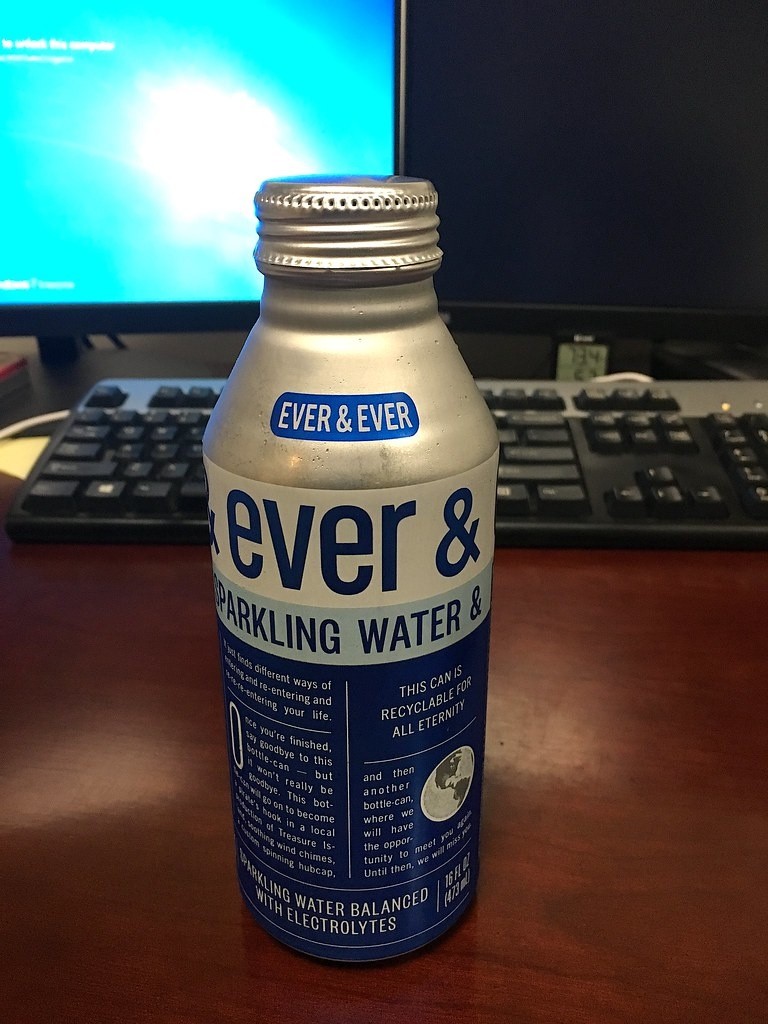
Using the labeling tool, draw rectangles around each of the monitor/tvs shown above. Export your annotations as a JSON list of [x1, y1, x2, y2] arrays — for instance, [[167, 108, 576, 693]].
[[0, 1, 768, 335]]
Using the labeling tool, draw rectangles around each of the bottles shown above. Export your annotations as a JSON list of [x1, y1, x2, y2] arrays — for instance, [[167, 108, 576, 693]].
[[199, 171, 502, 972]]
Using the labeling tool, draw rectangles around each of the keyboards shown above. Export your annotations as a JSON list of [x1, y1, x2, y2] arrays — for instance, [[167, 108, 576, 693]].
[[9, 374, 768, 549]]
